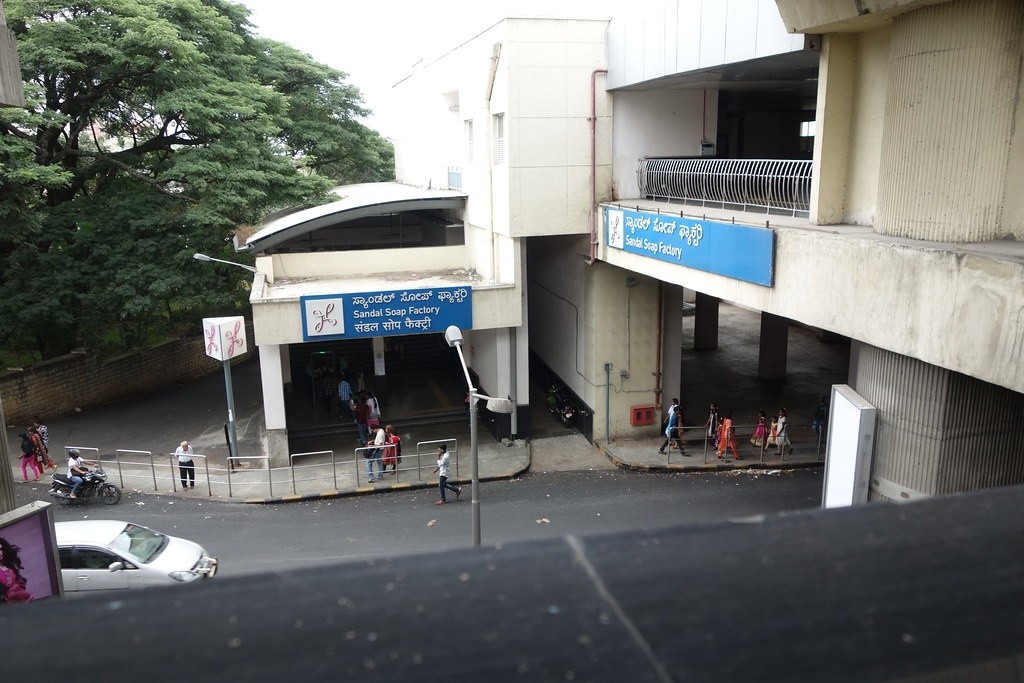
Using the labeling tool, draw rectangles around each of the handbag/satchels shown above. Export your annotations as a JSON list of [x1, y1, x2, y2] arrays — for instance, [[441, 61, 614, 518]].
[[71, 466, 88, 476], [367, 419, 380, 426], [362, 443, 377, 458]]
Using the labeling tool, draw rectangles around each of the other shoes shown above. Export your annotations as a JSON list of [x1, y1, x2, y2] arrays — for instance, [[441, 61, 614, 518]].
[[191, 486, 194, 488], [183, 488, 188, 491], [367, 478, 374, 483], [34, 478, 39, 481], [735, 456, 744, 460], [789, 448, 793, 455], [435, 500, 446, 504], [377, 475, 383, 480], [391, 472, 395, 475], [54, 465, 58, 472], [682, 454, 691, 457], [457, 487, 461, 498], [718, 456, 725, 459], [775, 452, 781, 455], [658, 451, 666, 455], [19, 479, 28, 483], [70, 494, 76, 498]]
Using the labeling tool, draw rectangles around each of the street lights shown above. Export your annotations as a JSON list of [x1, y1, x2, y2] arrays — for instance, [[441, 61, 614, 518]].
[[446, 324, 514, 548]]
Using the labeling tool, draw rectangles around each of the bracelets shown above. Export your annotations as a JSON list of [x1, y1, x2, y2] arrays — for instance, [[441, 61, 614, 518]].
[[93, 463, 95, 466]]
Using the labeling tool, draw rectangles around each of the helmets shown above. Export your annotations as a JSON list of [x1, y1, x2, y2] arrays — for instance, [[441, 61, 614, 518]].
[[69, 449, 80, 459]]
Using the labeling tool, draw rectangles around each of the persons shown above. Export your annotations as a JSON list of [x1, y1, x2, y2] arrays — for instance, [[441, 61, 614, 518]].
[[17, 432, 40, 484], [28, 427, 58, 474], [658, 398, 793, 459], [320, 369, 402, 483], [32, 419, 51, 470], [67, 449, 100, 498], [174, 441, 195, 491], [433, 444, 461, 505], [0, 538, 40, 604], [812, 394, 828, 446]]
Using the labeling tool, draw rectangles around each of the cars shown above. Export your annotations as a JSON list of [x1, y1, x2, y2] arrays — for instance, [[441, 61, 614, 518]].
[[54, 520, 217, 594]]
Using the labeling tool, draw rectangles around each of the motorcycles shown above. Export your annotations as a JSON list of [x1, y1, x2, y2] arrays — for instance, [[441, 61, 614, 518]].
[[547, 384, 575, 427], [49, 464, 121, 505]]
